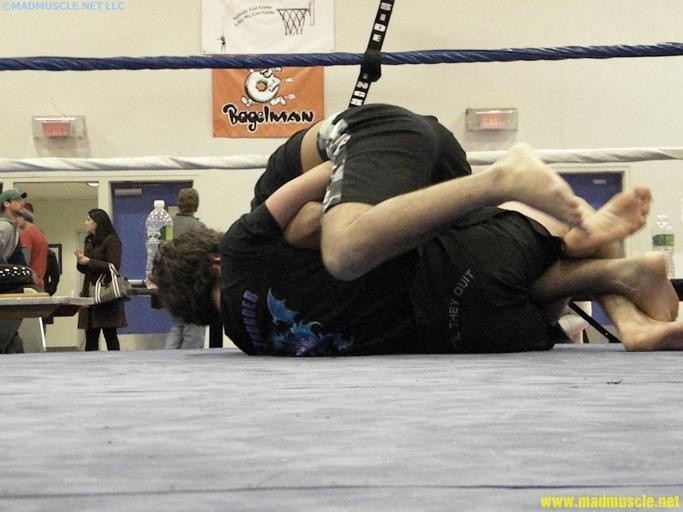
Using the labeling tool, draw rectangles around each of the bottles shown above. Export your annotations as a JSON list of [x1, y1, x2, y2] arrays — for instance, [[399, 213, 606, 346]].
[[651, 214, 674, 280], [143, 199, 174, 290]]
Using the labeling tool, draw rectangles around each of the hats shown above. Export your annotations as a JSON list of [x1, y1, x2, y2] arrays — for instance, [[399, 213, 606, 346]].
[[0, 190, 27, 205], [19, 204, 34, 223]]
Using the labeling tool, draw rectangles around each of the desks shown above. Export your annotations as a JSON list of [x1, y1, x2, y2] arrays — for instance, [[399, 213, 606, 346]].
[[127, 287, 223, 349], [1, 294, 92, 355]]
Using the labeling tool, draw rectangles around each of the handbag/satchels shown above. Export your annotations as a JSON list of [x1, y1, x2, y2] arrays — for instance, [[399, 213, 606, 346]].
[[88, 262, 131, 306]]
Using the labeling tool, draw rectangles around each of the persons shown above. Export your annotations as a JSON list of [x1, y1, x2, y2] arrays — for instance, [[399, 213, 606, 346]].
[[14, 202, 48, 293], [41, 247, 59, 335], [73, 208, 122, 351], [161, 185, 209, 350], [147, 159, 679, 357], [248, 101, 682, 353], [559, 300, 592, 344], [0, 188, 27, 355]]
